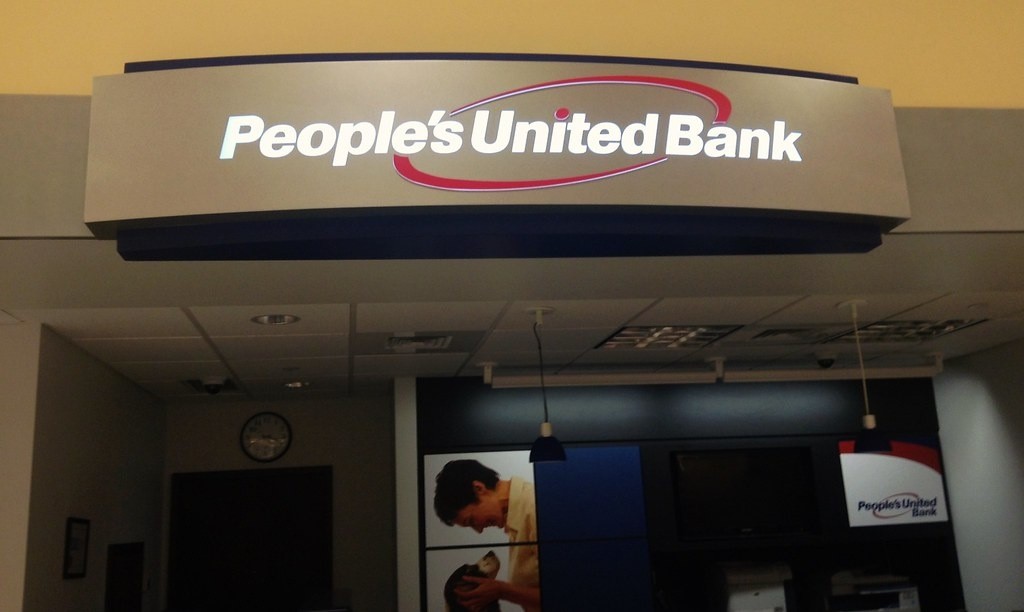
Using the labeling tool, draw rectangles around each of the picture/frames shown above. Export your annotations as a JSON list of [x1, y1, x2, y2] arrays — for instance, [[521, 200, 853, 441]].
[[62, 517, 90, 580]]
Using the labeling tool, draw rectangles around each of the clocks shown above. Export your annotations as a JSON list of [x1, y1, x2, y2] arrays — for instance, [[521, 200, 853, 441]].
[[240, 412, 292, 463]]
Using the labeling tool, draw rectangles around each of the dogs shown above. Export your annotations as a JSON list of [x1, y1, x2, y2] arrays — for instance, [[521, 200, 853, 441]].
[[444, 550, 501, 612]]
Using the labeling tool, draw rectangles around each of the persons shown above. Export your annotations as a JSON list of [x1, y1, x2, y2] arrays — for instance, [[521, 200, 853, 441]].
[[433, 460, 540, 611]]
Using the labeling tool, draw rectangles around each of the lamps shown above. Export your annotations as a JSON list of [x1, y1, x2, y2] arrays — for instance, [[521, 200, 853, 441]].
[[839, 299, 892, 454], [526, 306, 568, 464]]
